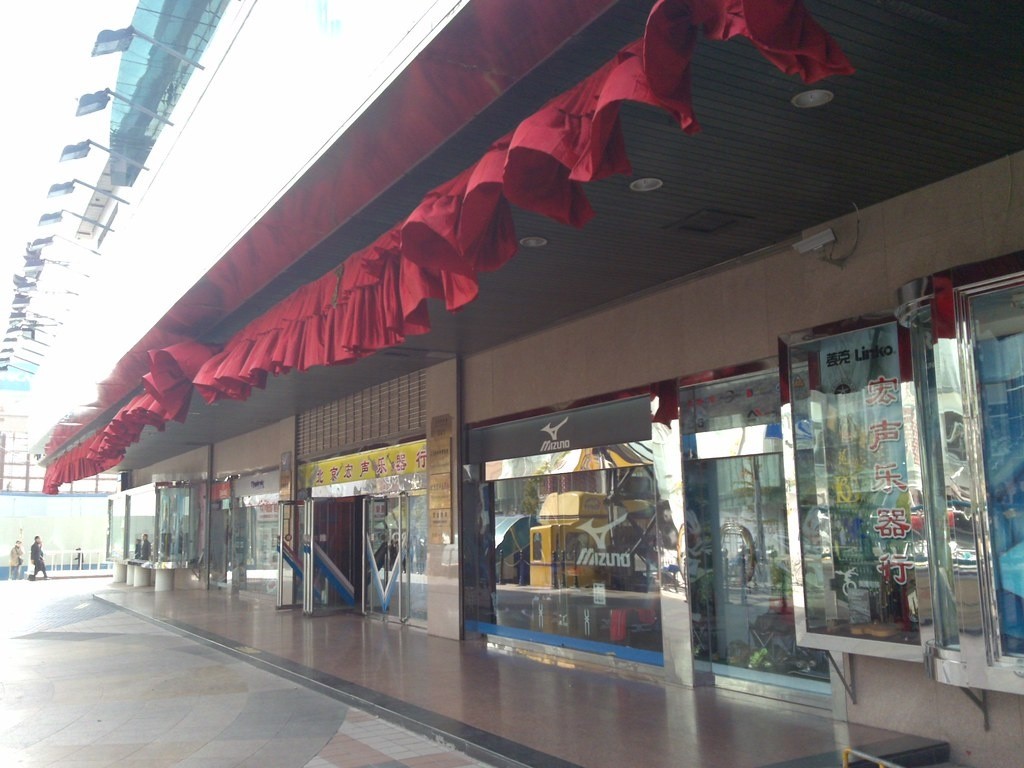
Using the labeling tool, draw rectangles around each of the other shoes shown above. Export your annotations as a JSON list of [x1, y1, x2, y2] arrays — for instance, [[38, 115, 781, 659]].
[[44, 577, 51, 580]]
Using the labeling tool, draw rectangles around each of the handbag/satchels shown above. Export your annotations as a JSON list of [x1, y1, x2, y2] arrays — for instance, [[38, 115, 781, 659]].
[[19, 558, 23, 565]]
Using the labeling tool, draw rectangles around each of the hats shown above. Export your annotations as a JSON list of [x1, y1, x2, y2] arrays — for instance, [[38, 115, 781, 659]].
[[76, 548, 81, 550]]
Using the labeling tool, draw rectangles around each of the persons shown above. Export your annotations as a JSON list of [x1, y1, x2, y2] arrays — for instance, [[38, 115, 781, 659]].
[[9, 540, 24, 580], [134, 539, 142, 559], [75, 548, 84, 570], [142, 534, 152, 561], [31, 536, 50, 580]]
